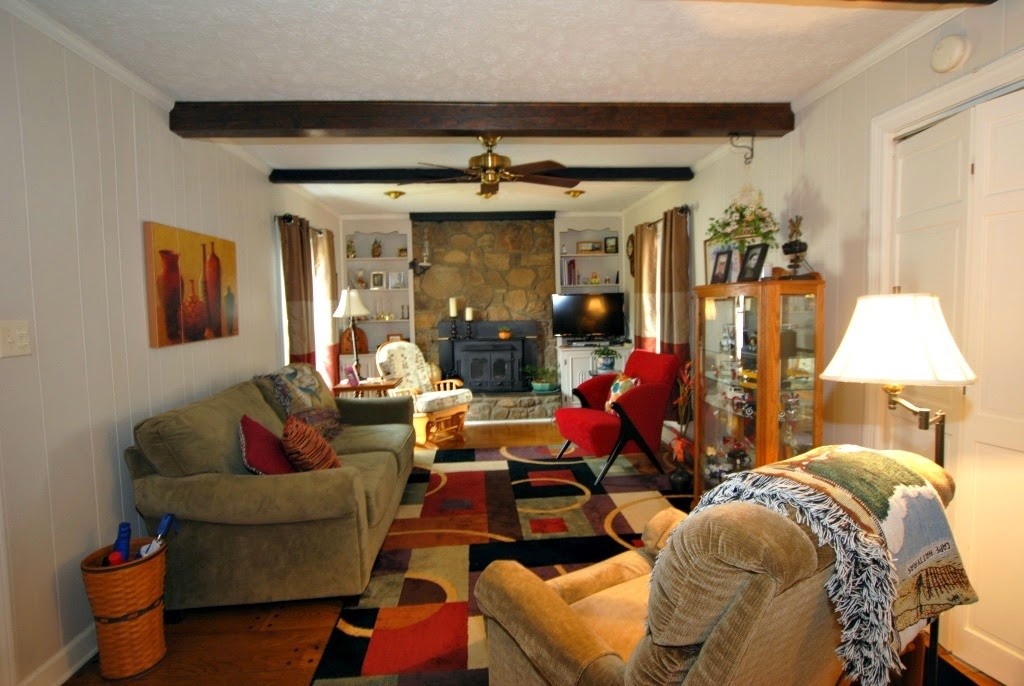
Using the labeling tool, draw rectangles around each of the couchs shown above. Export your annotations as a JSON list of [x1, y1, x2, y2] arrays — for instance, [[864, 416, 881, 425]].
[[132, 365, 416, 612]]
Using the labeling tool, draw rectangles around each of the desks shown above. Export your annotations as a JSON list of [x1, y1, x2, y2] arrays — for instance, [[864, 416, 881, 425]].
[[333, 377, 403, 398]]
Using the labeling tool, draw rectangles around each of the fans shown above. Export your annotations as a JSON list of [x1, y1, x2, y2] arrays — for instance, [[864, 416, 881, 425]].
[[417, 134, 579, 196]]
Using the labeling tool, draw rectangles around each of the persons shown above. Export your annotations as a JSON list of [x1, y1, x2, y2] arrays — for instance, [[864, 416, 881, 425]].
[[712, 252, 760, 283]]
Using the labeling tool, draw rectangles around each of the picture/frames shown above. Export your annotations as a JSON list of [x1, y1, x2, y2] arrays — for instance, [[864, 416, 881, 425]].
[[704, 239, 769, 284]]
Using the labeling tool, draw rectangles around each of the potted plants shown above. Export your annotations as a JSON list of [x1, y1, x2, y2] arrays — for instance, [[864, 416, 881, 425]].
[[594, 345, 616, 369], [527, 367, 559, 391], [498, 321, 510, 339]]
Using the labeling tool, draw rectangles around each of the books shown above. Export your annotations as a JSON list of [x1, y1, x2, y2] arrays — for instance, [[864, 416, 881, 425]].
[[561, 257, 577, 285]]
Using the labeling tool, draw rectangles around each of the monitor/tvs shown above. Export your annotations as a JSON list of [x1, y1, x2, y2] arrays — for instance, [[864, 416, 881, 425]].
[[551, 294, 625, 342]]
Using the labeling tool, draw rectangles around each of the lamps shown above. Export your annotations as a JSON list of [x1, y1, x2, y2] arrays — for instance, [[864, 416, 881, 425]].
[[333, 289, 370, 380], [820, 286, 978, 685]]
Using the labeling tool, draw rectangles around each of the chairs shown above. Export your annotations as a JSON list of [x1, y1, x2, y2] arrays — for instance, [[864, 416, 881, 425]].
[[554, 350, 676, 479], [476, 445, 954, 686], [376, 341, 471, 450]]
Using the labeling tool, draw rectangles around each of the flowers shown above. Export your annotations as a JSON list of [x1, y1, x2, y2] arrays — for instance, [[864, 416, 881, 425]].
[[708, 186, 781, 246]]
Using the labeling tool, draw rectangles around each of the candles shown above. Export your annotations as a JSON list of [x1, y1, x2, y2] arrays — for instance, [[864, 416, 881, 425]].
[[449, 297, 457, 316], [465, 307, 473, 320]]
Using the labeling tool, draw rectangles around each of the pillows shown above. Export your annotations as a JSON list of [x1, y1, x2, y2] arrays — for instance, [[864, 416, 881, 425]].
[[240, 363, 340, 475]]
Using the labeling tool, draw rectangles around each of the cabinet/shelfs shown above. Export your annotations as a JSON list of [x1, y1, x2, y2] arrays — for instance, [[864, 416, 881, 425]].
[[340, 217, 415, 400], [692, 275, 825, 509], [556, 213, 634, 407]]
